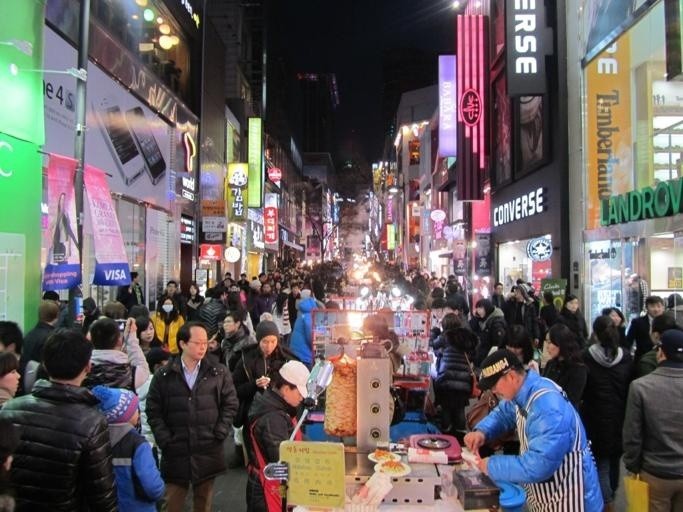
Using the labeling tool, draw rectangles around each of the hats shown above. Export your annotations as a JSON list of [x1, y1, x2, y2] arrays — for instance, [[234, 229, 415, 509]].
[[279, 359, 311, 400], [256, 320, 279, 341], [476, 350, 520, 390], [653, 329, 683, 353], [92, 384, 139, 424]]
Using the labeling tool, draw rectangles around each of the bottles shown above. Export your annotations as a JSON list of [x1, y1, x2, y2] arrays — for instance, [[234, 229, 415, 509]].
[[404, 329, 424, 336], [303, 359, 333, 409], [393, 306, 404, 327], [404, 314, 411, 328]]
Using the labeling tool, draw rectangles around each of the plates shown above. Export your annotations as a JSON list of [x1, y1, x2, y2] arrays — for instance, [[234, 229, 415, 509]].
[[373, 462, 412, 479], [366, 452, 401, 464]]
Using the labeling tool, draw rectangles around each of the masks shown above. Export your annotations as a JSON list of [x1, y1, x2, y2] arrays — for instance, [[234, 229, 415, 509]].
[[162, 304, 173, 312]]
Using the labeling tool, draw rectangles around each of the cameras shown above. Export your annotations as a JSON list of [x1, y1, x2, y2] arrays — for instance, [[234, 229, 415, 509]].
[[114, 319, 127, 332]]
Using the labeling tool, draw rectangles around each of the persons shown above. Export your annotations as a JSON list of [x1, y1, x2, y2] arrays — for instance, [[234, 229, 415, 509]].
[[1, 251, 683, 511]]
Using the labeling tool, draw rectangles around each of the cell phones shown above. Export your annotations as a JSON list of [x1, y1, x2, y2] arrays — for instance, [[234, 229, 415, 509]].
[[91, 96, 145, 186], [125, 107, 166, 185]]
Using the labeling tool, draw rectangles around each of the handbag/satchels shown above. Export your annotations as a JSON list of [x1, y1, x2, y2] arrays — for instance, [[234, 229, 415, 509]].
[[623, 475, 649, 512], [466, 390, 502, 450]]
